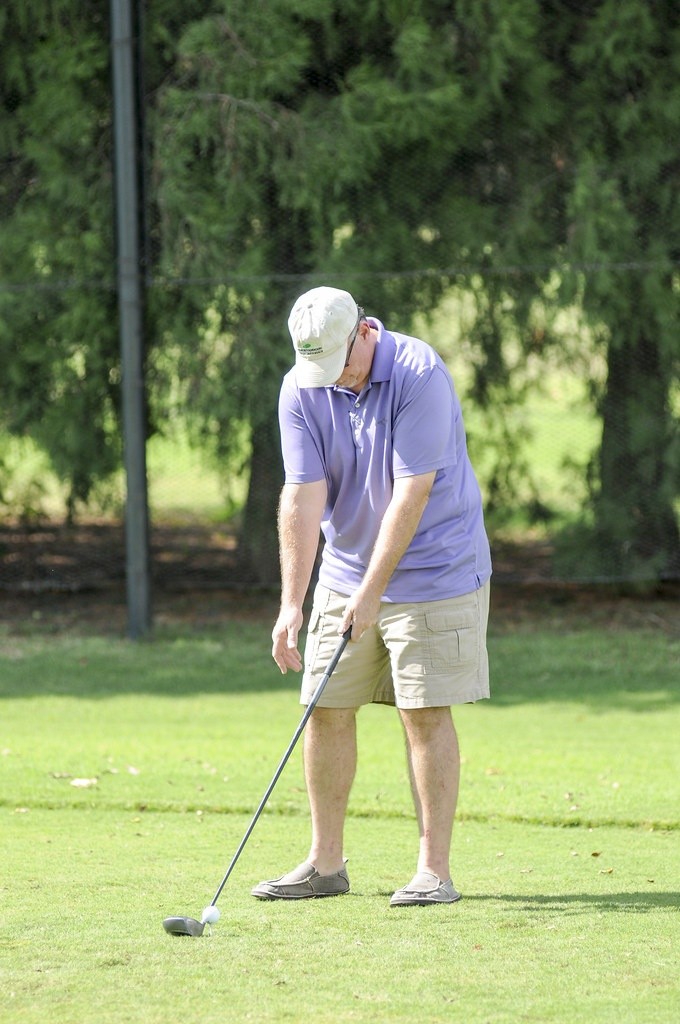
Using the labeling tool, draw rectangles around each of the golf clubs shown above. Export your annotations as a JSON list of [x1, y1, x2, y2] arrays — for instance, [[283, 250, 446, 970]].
[[161, 623, 354, 939]]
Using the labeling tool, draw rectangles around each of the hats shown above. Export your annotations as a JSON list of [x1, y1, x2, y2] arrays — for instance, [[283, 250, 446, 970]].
[[287, 286, 359, 389]]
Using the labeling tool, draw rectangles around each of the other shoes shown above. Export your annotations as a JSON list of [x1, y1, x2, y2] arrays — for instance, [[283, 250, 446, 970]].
[[250, 859, 349, 898], [389, 872, 462, 907]]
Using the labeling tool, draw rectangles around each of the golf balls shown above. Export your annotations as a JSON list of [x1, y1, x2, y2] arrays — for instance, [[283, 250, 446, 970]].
[[202, 906, 220, 923]]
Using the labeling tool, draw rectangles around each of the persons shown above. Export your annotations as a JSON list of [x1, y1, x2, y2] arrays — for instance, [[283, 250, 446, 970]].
[[251, 286, 493, 908]]
